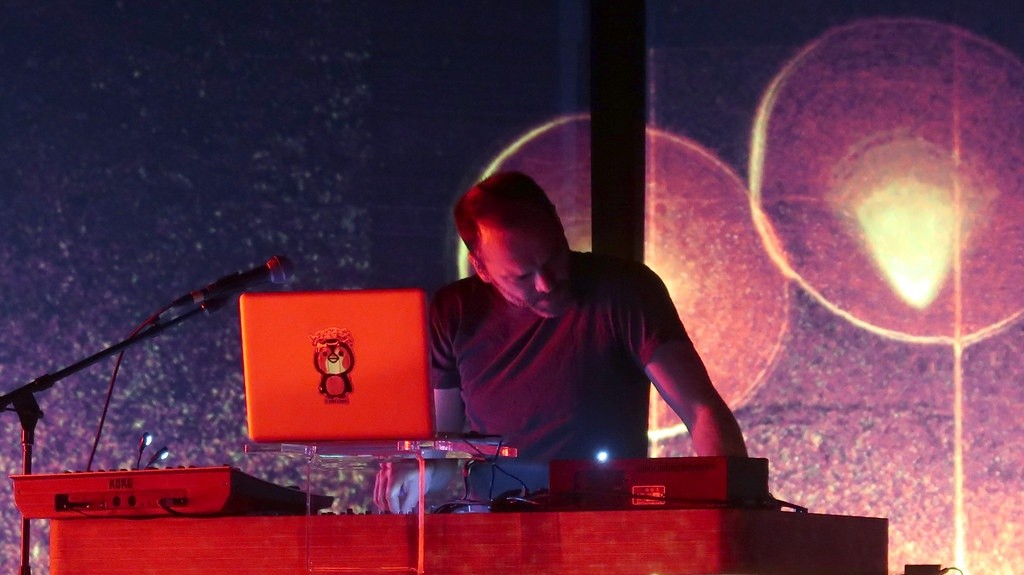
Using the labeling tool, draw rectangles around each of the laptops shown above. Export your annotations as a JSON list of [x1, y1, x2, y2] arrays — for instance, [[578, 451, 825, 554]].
[[239, 288, 511, 447]]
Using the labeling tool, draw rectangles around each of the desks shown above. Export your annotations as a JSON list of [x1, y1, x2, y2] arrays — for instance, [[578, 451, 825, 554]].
[[49, 509, 890, 575]]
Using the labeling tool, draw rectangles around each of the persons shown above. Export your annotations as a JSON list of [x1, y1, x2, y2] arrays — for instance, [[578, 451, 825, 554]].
[[373, 171, 774, 513]]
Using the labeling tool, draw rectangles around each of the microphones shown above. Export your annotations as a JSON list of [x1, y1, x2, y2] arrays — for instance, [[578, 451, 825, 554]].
[[177, 255, 294, 310]]
